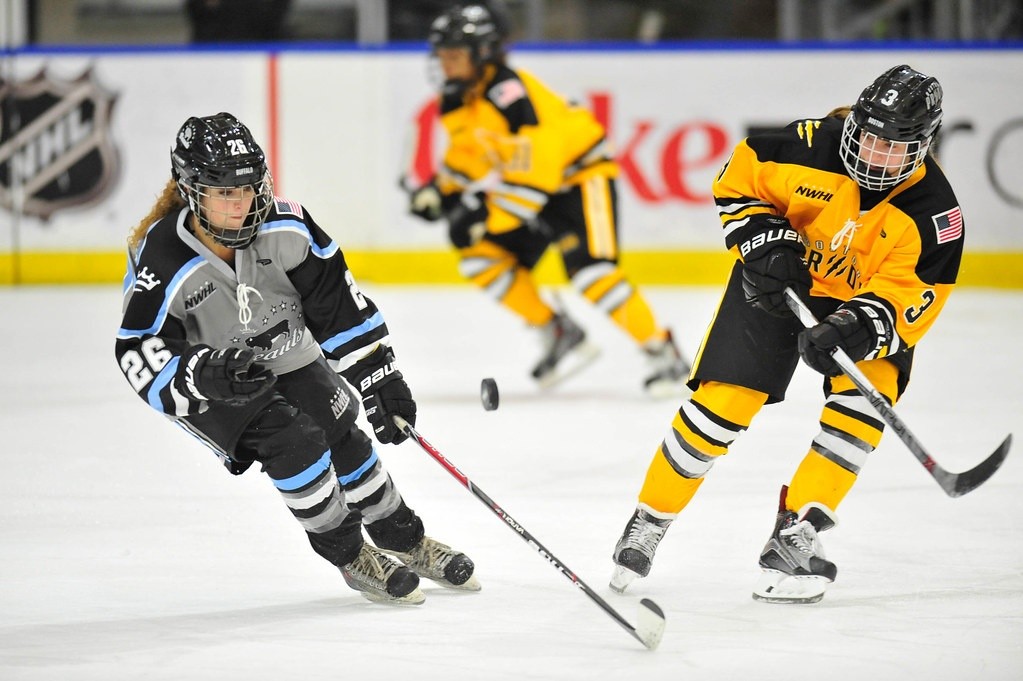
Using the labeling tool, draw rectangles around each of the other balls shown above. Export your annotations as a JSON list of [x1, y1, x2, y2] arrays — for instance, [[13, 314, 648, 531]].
[[478, 375, 505, 410]]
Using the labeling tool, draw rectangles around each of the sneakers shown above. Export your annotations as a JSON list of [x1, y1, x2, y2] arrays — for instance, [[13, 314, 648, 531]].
[[609, 502, 677, 593], [644, 329, 690, 399], [752, 501, 840, 605], [338, 541, 425, 602], [396, 535, 481, 591], [530, 312, 592, 391]]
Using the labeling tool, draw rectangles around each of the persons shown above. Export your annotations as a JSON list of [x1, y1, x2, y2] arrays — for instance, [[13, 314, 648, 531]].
[[400, 7, 692, 400], [611, 65, 964, 605], [116, 113, 482, 603]]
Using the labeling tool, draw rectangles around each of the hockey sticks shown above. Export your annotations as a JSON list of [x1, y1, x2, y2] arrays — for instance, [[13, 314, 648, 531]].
[[393, 418, 669, 652], [787, 282, 1014, 497]]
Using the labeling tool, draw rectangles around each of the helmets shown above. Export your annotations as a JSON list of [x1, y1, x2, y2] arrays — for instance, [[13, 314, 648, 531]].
[[170, 112, 274, 250], [428, 4, 509, 95], [838, 64, 943, 191]]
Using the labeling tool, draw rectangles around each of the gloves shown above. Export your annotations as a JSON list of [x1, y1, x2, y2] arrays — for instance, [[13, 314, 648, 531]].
[[736, 214, 813, 318], [449, 192, 489, 248], [411, 183, 444, 220], [346, 343, 417, 445], [798, 292, 908, 378], [176, 344, 278, 401]]
